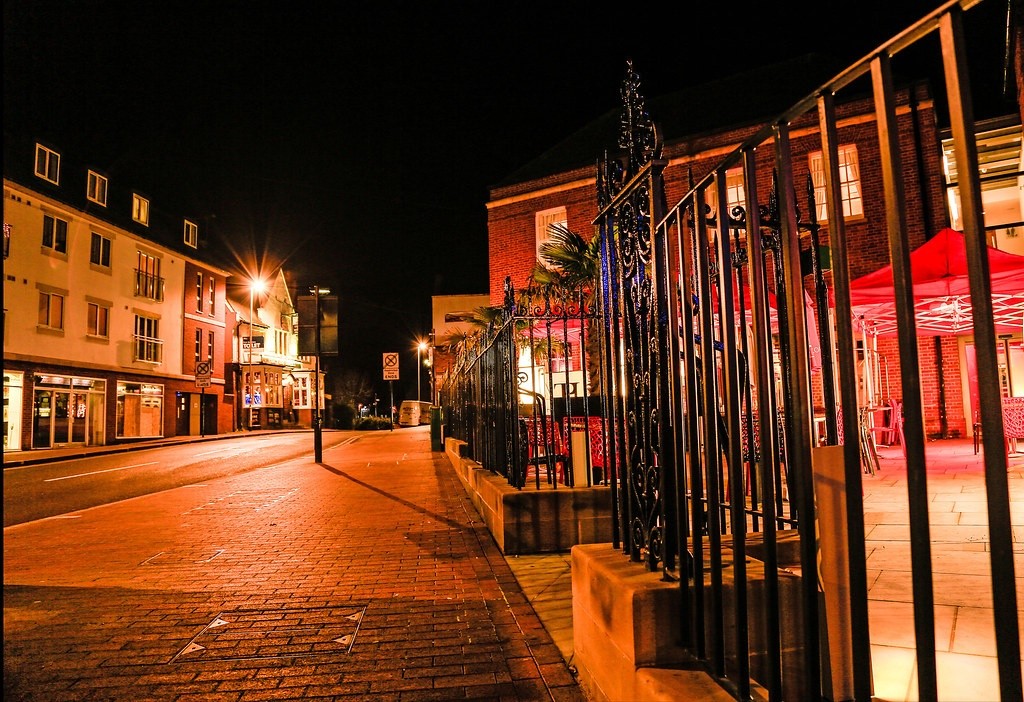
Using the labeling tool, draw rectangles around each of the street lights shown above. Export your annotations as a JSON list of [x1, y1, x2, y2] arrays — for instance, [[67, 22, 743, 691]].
[[417, 341, 428, 400], [248, 279, 267, 431], [309, 284, 331, 463]]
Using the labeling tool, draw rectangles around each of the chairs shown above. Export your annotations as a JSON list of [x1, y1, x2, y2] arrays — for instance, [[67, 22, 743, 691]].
[[972, 409, 983, 454], [1002, 397, 1024, 449], [528, 412, 623, 484], [869, 399, 904, 452]]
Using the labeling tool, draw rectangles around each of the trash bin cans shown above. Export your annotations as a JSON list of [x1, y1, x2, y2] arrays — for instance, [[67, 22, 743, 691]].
[[420, 401, 433, 425], [399, 400, 421, 426]]
